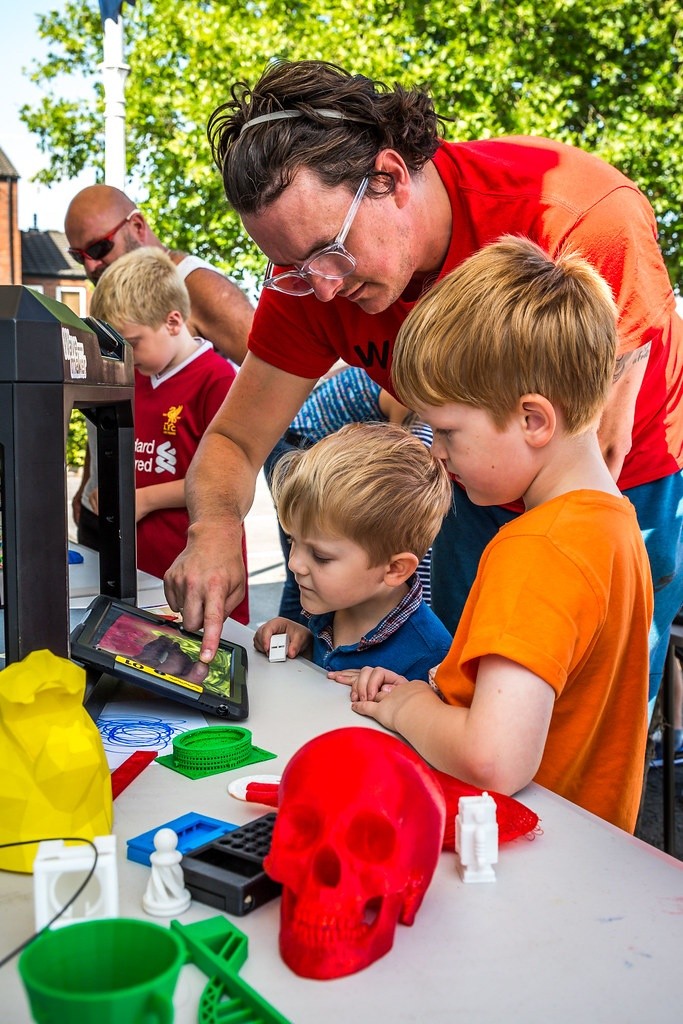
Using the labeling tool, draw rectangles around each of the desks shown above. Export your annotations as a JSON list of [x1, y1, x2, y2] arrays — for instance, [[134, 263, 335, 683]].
[[0, 541, 683, 1023]]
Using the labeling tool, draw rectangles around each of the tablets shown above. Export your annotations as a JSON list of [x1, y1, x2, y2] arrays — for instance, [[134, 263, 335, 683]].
[[70, 595, 248, 722]]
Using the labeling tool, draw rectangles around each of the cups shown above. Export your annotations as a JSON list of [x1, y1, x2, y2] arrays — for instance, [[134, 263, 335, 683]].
[[18, 918, 188, 1024]]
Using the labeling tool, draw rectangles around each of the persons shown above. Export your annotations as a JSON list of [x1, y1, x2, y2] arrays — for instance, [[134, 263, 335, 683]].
[[324, 231, 657, 836], [252, 420, 455, 685], [63, 184, 257, 552], [86, 244, 251, 629], [163, 57, 683, 779]]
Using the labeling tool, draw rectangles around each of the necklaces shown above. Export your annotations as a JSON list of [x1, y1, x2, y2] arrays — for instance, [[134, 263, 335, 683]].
[[166, 248, 171, 255]]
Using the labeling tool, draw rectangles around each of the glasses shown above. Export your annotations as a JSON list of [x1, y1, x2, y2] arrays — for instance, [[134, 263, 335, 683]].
[[263, 170, 372, 297], [67, 210, 142, 265]]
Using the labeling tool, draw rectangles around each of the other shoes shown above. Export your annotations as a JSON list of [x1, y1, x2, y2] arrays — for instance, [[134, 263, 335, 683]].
[[647, 725, 683, 767]]
